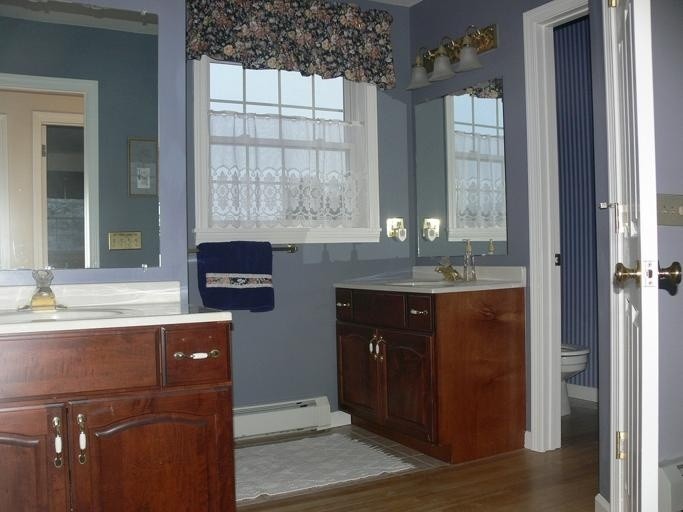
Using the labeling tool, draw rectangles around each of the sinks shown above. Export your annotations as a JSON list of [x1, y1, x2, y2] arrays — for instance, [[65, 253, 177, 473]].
[[384, 280, 460, 291], [0, 308, 125, 328]]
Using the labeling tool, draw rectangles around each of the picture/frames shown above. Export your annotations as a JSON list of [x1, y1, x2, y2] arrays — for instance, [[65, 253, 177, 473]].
[[126, 137, 157, 198]]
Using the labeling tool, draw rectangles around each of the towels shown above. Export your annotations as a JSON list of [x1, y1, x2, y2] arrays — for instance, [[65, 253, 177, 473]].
[[197, 241, 275, 314]]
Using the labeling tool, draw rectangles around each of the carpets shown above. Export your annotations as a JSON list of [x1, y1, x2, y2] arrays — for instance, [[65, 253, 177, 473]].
[[235, 435, 413, 505]]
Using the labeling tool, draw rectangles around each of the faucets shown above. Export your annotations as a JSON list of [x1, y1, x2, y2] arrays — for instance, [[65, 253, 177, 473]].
[[29, 269, 58, 311], [434, 263, 462, 282]]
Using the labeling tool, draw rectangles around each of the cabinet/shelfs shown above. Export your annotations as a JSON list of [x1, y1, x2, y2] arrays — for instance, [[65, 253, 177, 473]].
[[333, 266, 526, 464], [0, 313, 235, 511]]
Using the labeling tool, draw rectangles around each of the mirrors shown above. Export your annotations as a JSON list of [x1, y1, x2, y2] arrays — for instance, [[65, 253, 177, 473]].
[[1, 74, 99, 270], [412, 80, 507, 255]]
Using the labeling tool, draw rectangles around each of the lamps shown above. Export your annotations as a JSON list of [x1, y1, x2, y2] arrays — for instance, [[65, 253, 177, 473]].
[[454, 25, 484, 72], [428, 35, 459, 83], [406, 45, 431, 90]]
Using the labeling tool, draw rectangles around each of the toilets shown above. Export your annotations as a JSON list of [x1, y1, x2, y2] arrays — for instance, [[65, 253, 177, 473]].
[[560, 343, 590, 417]]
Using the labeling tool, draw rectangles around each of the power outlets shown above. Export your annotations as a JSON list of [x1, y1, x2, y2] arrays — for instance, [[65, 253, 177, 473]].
[[422, 218, 438, 238], [109, 232, 143, 250], [386, 217, 405, 238], [657, 194, 683, 226]]
